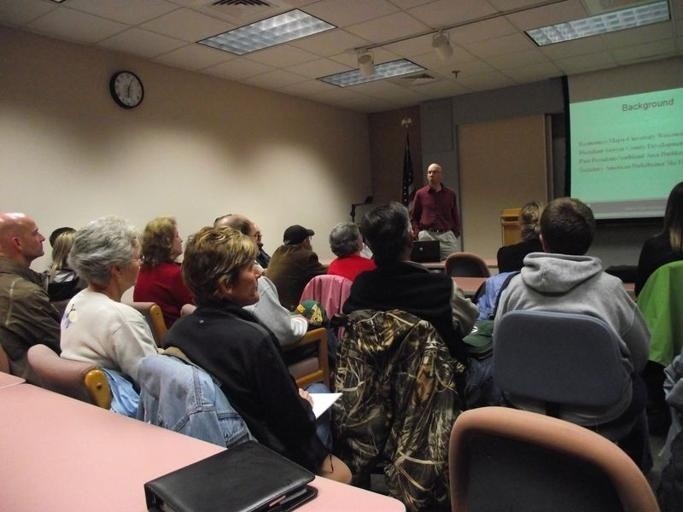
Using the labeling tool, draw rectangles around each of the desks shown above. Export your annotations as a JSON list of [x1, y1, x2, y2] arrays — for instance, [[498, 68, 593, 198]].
[[0, 371, 26, 389], [0, 383, 406, 512]]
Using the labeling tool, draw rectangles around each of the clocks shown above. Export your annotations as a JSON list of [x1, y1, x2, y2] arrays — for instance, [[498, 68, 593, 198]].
[[110, 70, 144, 109]]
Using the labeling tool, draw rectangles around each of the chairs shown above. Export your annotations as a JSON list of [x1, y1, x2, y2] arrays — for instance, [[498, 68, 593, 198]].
[[28, 252, 659, 512]]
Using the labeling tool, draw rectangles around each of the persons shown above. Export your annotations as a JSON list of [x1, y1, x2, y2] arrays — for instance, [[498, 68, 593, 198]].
[[0, 213, 194, 393], [519, 199, 550, 239], [216, 201, 480, 388], [408, 162, 461, 260], [489, 197, 654, 476], [633, 180, 683, 300], [160, 224, 331, 475]]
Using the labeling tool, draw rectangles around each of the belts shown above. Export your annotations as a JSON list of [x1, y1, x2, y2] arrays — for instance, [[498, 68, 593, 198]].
[[426, 227, 449, 233]]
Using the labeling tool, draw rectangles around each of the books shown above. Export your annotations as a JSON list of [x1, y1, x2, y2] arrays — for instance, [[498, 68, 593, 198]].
[[141, 437, 317, 511]]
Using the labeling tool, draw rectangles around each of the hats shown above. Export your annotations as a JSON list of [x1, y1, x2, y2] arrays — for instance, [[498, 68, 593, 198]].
[[462, 319, 494, 359], [291, 300, 328, 323], [284, 225, 314, 245]]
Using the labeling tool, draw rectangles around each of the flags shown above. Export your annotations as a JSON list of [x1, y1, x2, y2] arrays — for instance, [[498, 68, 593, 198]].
[[401, 127, 416, 207]]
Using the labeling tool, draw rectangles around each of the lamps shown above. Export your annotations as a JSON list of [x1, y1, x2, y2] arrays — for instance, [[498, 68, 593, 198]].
[[432, 26, 452, 60], [356, 48, 375, 77]]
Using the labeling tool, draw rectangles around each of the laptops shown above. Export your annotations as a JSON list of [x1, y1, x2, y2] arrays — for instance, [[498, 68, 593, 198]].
[[412, 240, 446, 263]]
